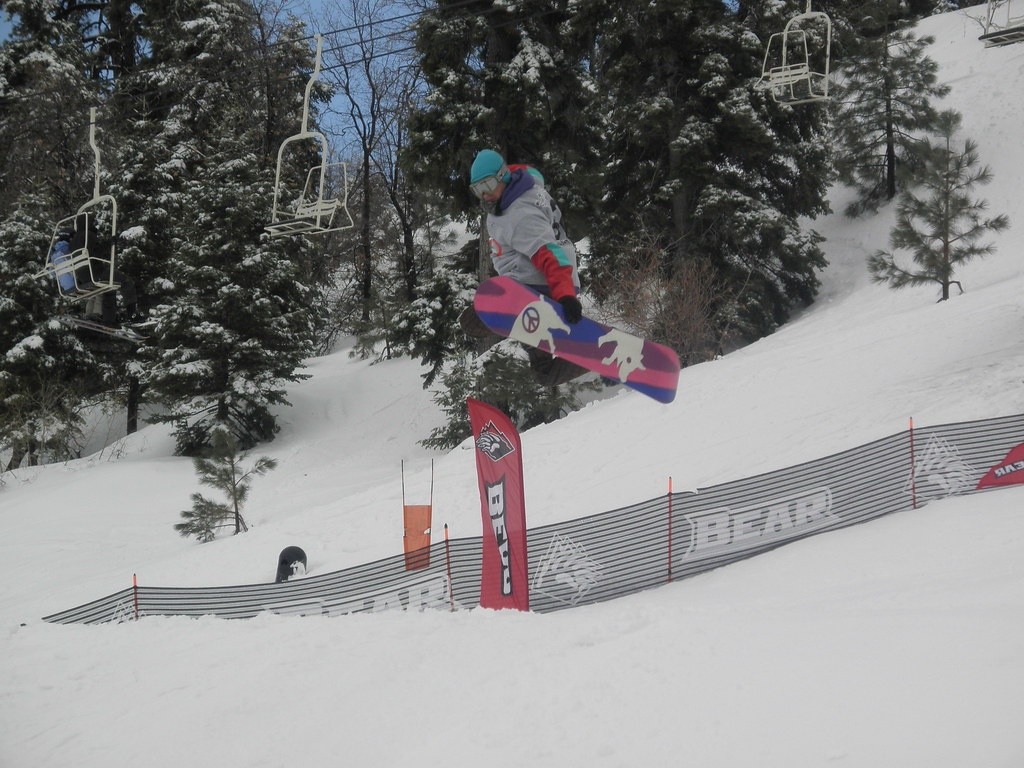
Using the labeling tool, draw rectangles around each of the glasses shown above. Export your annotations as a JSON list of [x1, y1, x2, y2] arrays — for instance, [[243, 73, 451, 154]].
[[469, 176, 499, 199]]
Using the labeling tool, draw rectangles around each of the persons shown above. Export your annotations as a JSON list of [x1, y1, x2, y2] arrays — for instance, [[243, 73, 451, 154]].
[[50, 214, 149, 331], [457, 149, 620, 388]]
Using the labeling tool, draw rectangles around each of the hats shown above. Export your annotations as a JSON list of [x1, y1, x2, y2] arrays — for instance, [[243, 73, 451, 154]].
[[470, 149, 511, 184]]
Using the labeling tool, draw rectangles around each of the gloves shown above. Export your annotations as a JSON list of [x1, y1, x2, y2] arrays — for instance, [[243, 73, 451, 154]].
[[558, 295, 583, 324]]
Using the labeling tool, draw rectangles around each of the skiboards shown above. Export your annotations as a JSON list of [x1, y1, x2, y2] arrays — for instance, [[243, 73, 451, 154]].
[[48, 312, 150, 343], [128, 320, 157, 328]]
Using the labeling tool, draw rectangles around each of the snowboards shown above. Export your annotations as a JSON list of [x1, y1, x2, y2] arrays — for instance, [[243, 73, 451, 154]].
[[472, 277, 683, 407]]
